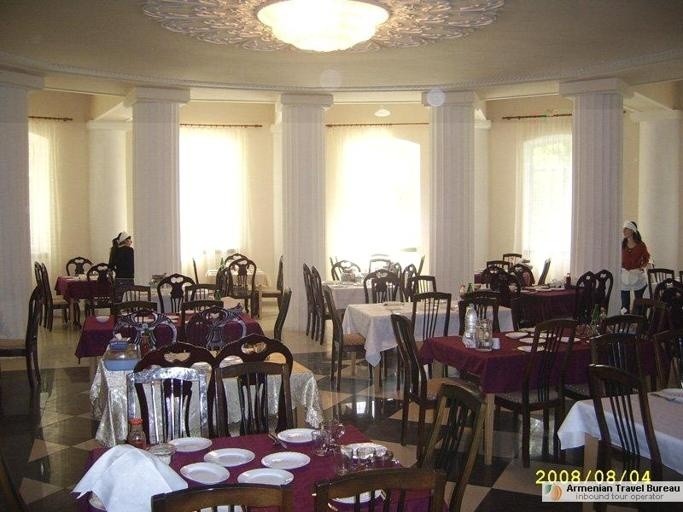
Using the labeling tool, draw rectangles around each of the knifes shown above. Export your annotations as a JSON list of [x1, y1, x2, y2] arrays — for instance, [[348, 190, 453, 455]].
[[267, 432, 288, 449]]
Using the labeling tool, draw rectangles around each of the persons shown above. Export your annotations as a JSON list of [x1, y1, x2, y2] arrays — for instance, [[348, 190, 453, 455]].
[[105, 230, 134, 287], [619, 220, 650, 315]]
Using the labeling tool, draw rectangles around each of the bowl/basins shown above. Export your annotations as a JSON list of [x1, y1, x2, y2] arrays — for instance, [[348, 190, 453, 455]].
[[96, 316, 110, 323]]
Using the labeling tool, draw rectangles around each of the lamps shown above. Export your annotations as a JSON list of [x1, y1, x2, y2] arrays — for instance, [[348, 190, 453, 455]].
[[373, 104, 391, 117], [253, 0, 394, 55]]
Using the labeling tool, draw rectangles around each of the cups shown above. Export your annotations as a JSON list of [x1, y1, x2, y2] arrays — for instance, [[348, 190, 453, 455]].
[[312, 418, 394, 477], [150, 444, 176, 464]]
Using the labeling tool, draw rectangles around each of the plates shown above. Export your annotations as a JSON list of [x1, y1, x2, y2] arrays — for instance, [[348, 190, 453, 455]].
[[62, 274, 98, 281], [122, 310, 199, 324], [88, 497, 107, 512], [510, 273, 571, 293], [278, 428, 319, 443], [506, 332, 581, 352], [169, 437, 310, 487], [383, 300, 459, 311]]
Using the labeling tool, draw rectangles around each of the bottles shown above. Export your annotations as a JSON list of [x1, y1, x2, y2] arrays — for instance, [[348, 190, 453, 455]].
[[462, 304, 500, 351], [460, 283, 474, 306], [140, 324, 154, 360], [593, 303, 606, 334], [323, 268, 371, 287], [127, 418, 146, 450]]
